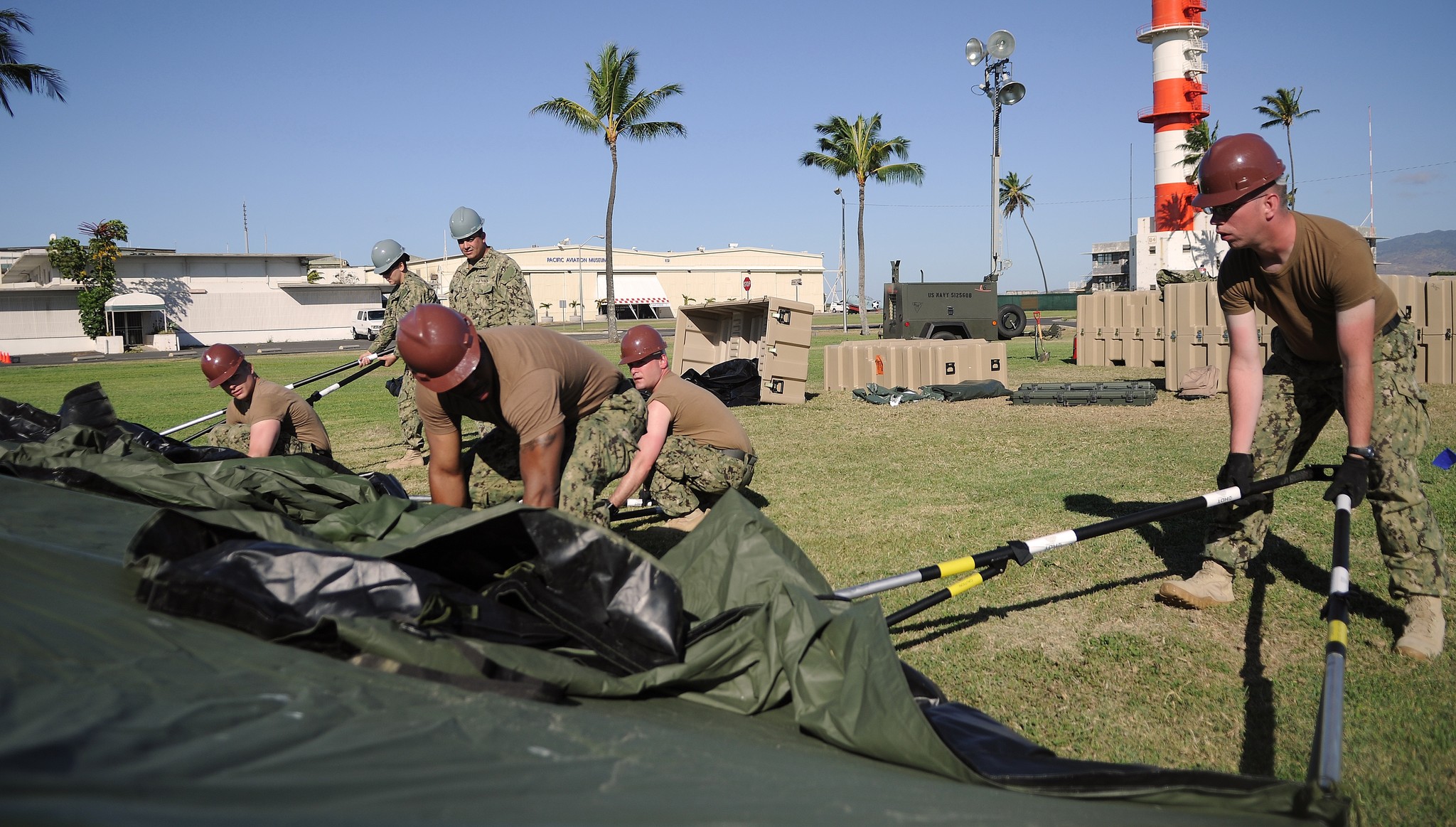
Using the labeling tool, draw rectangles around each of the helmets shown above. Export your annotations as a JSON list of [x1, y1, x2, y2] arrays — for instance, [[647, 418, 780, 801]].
[[372, 239, 405, 274], [395, 303, 480, 392], [617, 324, 667, 365], [1191, 133, 1286, 209], [449, 206, 485, 239], [201, 342, 244, 388]]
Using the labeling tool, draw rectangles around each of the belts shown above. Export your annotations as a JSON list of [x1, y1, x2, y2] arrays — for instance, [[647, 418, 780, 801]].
[[1381, 313, 1401, 335], [317, 449, 332, 456], [610, 378, 631, 397], [722, 448, 757, 465]]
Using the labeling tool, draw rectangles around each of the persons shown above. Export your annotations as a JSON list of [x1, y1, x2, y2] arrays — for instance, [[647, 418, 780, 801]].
[[1161, 133, 1450, 663], [449, 207, 536, 438], [583, 324, 758, 533], [396, 304, 650, 519], [359, 239, 442, 468], [200, 343, 333, 457]]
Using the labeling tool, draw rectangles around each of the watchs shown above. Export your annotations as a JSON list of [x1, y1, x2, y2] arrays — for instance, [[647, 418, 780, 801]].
[[1347, 446, 1375, 458], [606, 499, 619, 516]]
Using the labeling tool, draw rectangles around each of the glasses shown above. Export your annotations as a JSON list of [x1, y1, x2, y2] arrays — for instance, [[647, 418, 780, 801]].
[[1202, 193, 1268, 216]]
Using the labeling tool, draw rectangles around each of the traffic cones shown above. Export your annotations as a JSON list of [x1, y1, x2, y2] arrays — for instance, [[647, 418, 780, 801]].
[[5, 352, 12, 363], [1, 352, 7, 363], [0, 351, 3, 362]]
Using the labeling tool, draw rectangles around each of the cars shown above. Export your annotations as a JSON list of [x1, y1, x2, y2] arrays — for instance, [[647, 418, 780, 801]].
[[830, 300, 849, 313]]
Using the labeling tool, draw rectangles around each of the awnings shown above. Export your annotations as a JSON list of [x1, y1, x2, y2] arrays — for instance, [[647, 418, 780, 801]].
[[597, 275, 669, 304]]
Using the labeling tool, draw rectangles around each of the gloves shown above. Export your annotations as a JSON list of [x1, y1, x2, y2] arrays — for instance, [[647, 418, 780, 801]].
[[1322, 454, 1369, 509], [1216, 451, 1267, 507]]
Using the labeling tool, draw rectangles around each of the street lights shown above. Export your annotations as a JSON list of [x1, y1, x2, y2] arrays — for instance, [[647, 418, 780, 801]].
[[834, 187, 849, 333], [579, 235, 605, 331], [964, 30, 1027, 281]]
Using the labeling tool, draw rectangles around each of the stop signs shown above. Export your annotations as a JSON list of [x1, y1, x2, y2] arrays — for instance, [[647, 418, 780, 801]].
[[744, 277, 751, 291]]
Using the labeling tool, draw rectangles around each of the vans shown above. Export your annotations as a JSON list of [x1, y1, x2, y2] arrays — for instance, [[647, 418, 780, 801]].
[[351, 308, 391, 341]]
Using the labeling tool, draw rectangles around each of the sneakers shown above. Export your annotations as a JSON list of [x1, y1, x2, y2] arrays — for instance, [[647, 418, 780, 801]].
[[655, 507, 710, 536], [1159, 559, 1235, 609], [1391, 592, 1445, 662]]
